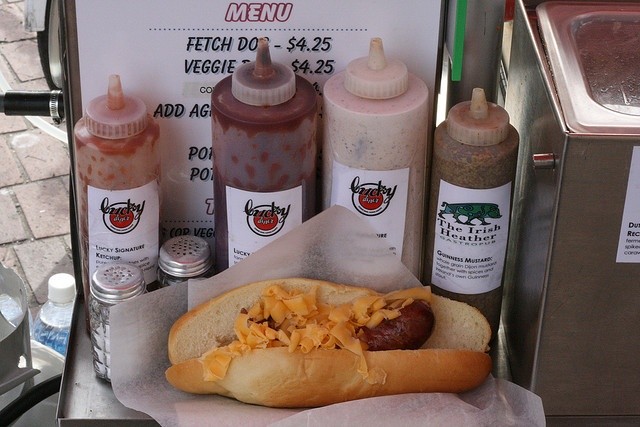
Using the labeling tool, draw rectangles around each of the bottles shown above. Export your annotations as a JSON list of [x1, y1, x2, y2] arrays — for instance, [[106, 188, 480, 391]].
[[159, 234, 213, 292], [89, 261, 149, 383], [73, 75, 164, 333], [212, 38, 319, 278], [322, 35, 429, 287], [423, 87, 520, 341], [29, 274, 77, 360]]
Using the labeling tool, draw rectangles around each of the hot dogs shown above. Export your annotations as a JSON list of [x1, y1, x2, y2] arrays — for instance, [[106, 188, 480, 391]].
[[167, 278, 493, 408]]
